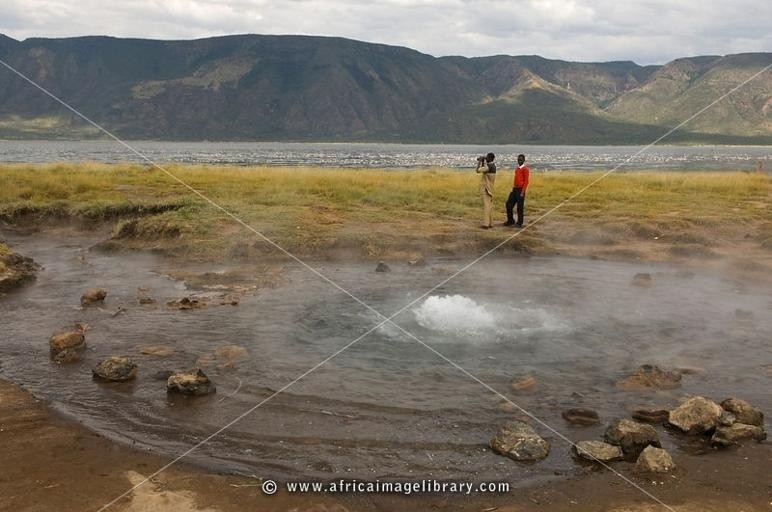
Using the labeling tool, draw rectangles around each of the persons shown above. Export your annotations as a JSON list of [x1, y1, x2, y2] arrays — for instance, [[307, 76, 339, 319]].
[[475, 152, 496, 229], [504, 154, 529, 228]]
